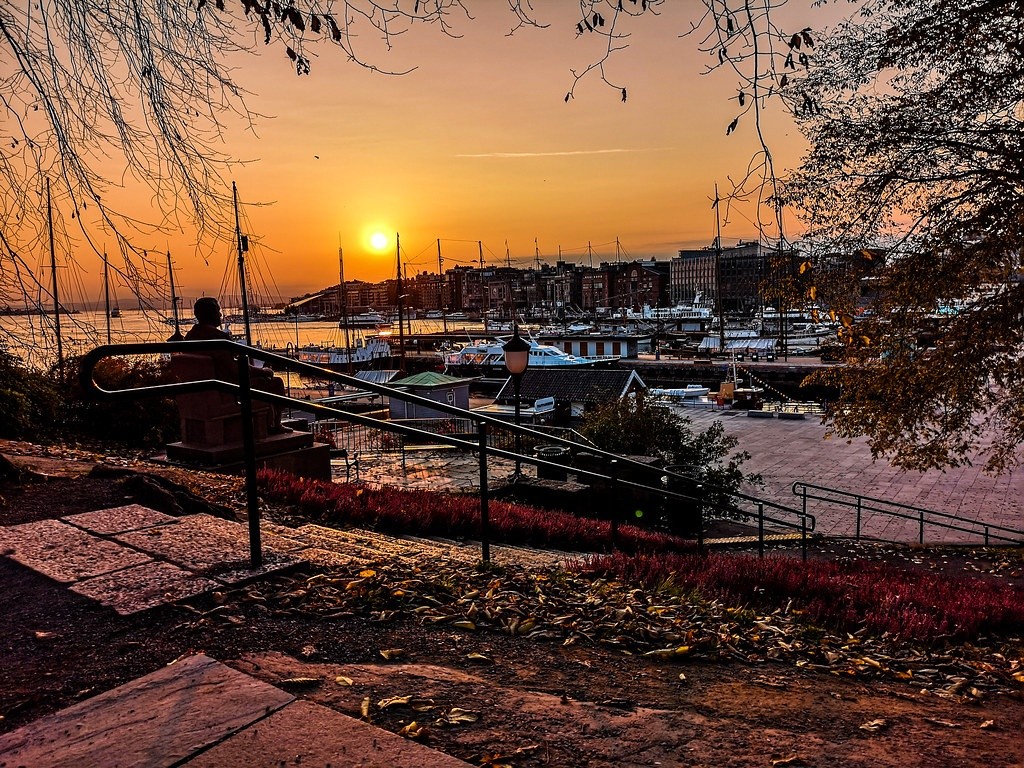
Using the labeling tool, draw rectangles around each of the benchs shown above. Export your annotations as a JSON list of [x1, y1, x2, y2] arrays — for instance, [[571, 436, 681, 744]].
[[402, 434, 478, 465], [330, 448, 359, 481]]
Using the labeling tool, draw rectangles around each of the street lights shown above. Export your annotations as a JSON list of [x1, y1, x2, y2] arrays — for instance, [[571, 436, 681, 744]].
[[501, 322, 532, 482]]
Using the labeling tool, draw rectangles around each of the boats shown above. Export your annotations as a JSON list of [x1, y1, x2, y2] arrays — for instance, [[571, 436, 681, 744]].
[[111, 306, 123, 318]]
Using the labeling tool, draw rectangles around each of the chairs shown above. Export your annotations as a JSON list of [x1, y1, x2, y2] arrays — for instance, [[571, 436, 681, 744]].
[[173, 353, 275, 444]]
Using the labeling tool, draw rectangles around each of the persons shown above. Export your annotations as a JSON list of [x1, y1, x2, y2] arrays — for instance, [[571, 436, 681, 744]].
[[184, 297, 293, 431]]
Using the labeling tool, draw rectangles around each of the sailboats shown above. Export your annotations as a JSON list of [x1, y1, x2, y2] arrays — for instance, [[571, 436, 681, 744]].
[[166, 181, 889, 420]]
